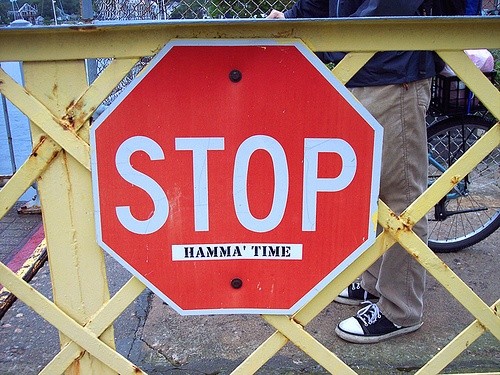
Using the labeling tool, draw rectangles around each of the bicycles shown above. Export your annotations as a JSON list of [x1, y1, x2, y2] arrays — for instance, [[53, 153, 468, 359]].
[[250, 12, 499, 252]]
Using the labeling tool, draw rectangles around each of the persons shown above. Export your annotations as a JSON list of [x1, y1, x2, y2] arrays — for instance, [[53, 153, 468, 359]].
[[264, 0, 436, 344]]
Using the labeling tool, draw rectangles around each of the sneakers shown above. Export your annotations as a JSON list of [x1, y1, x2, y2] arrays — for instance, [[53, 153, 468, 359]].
[[334, 301, 423, 344], [334, 281, 380, 305]]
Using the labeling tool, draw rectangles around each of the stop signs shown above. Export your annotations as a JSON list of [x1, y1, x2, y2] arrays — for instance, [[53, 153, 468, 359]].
[[89, 37, 384, 315]]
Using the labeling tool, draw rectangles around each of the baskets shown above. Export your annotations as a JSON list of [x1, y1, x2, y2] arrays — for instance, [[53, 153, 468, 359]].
[[432, 70, 497, 109]]
